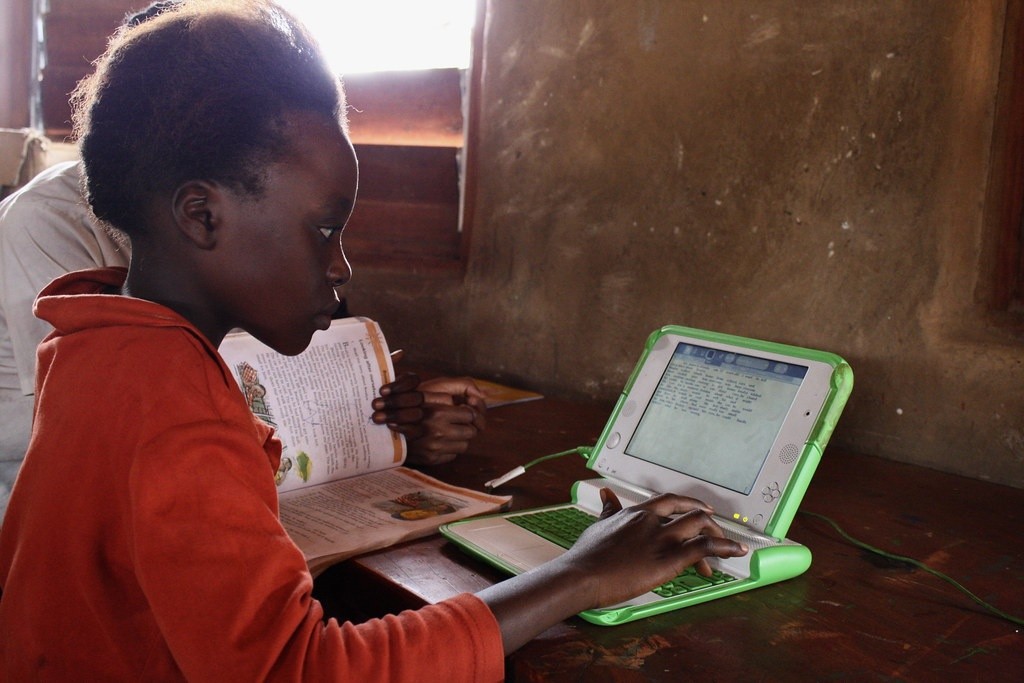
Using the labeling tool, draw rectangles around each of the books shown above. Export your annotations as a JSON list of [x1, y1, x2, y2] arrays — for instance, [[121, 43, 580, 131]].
[[473, 377, 544, 409], [217, 316, 514, 582]]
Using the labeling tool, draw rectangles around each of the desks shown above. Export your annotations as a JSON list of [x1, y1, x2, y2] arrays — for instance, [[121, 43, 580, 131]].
[[346, 363, 1024, 683]]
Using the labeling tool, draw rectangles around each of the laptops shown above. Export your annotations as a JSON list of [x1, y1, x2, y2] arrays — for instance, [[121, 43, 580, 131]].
[[438, 324, 853, 624]]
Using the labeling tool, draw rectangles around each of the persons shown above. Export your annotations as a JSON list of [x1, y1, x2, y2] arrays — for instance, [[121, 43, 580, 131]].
[[1, 0, 748, 683], [1, 0, 490, 526]]
